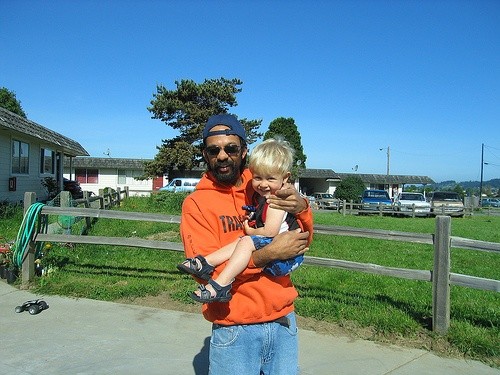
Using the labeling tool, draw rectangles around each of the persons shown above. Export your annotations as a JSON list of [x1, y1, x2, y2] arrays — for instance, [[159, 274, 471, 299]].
[[176, 138, 303, 304], [179, 114, 314, 375]]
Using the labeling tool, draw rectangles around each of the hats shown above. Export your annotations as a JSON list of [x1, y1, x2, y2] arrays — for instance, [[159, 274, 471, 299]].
[[203, 115, 247, 144]]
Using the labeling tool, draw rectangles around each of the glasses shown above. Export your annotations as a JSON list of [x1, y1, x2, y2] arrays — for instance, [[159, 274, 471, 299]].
[[204, 145, 241, 157]]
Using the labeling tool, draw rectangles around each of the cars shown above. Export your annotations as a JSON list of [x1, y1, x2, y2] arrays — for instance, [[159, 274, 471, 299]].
[[480, 198, 500, 207], [310, 192, 343, 210], [15, 297, 47, 315]]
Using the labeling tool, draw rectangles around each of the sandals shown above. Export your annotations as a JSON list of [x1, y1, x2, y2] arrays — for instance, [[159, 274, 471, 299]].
[[190, 279, 232, 303], [176, 255, 215, 281]]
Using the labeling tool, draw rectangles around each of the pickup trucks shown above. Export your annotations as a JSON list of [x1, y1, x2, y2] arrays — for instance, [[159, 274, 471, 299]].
[[393, 192, 431, 217], [427, 190, 464, 218], [358, 189, 393, 215]]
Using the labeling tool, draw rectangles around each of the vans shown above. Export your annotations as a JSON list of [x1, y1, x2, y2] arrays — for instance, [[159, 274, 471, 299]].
[[159, 177, 201, 194]]
